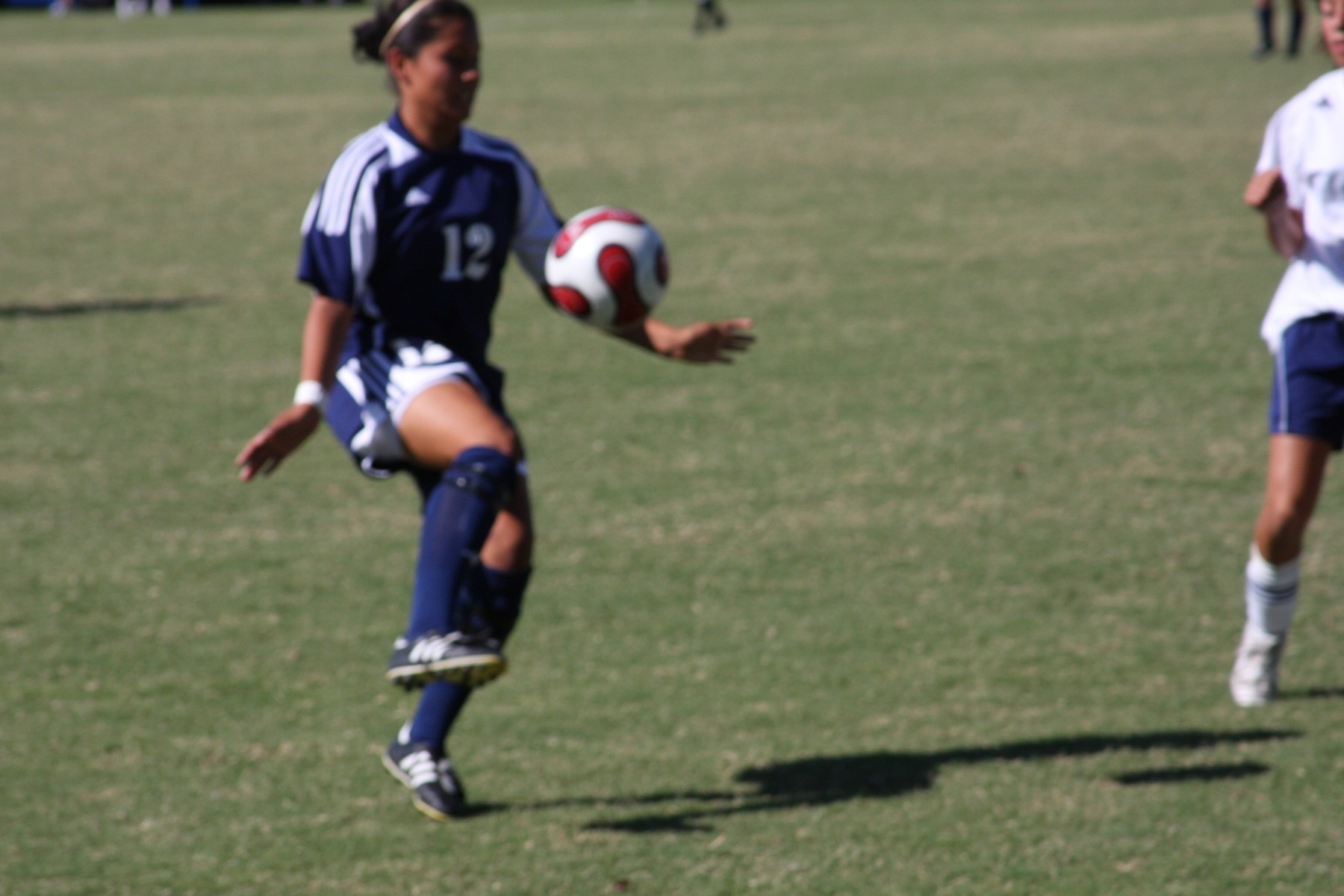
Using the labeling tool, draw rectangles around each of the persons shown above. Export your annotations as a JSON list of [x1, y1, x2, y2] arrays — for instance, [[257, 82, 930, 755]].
[[1227, 0, 1343, 708], [1250, 0, 1307, 60], [234, 0, 757, 820]]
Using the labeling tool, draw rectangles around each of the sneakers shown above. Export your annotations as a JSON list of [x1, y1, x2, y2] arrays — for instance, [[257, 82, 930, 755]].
[[385, 628, 504, 689], [1230, 623, 1285, 707], [382, 721, 466, 823]]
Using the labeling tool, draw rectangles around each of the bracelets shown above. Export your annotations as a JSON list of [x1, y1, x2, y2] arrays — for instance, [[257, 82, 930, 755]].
[[294, 379, 329, 417]]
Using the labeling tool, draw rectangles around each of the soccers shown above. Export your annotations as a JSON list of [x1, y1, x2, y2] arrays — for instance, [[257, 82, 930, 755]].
[[543, 205, 671, 334]]
